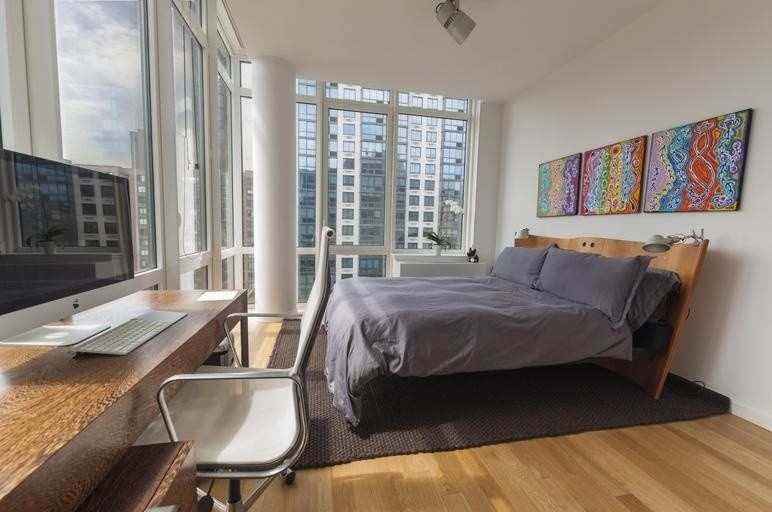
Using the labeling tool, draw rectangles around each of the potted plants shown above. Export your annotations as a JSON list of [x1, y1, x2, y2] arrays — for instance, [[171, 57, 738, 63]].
[[428, 231, 448, 256]]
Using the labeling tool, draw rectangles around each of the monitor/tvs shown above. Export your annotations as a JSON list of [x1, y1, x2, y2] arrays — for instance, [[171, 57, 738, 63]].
[[0, 148, 135, 347]]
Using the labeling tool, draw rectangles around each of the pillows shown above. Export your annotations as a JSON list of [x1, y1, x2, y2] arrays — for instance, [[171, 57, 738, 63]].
[[534, 246, 652, 330], [490, 242, 558, 287]]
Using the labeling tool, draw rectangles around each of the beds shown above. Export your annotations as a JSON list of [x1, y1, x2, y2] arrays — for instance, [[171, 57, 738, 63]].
[[321, 235, 710, 427]]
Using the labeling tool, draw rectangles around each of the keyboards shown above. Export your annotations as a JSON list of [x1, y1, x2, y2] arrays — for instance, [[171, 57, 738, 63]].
[[66, 310, 188, 358]]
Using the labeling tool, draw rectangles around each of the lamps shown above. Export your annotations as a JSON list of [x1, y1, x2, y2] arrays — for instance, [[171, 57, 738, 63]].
[[515, 228, 528, 239], [435, 0, 477, 45], [643, 230, 704, 252]]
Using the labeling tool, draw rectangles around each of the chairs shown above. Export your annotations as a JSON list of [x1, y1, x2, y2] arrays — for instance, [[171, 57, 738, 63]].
[[157, 227, 335, 511]]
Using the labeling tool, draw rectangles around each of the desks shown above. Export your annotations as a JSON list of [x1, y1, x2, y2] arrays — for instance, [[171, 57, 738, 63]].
[[0, 289, 249, 512], [392, 254, 487, 278]]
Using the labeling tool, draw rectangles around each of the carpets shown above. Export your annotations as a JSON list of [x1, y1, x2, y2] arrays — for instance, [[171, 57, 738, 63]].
[[268, 317, 730, 468]]
